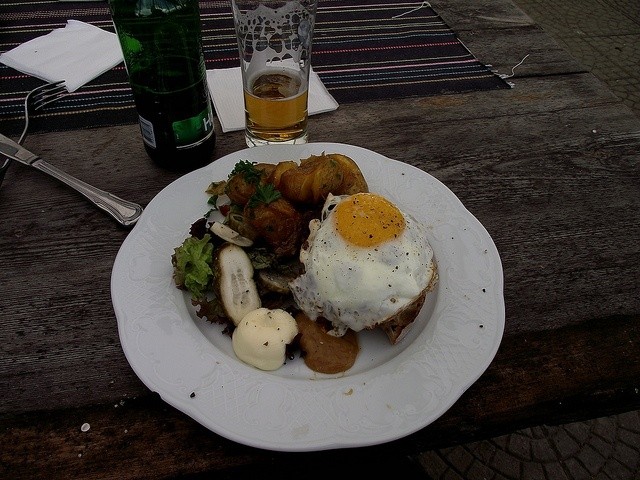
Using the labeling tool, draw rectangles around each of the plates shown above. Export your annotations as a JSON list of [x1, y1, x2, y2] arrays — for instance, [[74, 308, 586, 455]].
[[111, 142, 505, 451]]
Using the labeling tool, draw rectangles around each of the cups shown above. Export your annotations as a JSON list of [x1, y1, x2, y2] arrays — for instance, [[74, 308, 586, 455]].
[[233, 0, 316, 147]]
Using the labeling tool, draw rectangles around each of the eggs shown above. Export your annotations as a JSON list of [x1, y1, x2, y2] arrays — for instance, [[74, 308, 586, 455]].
[[302, 192, 435, 328]]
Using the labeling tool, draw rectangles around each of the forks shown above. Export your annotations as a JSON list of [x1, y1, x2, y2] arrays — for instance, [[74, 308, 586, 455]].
[[1, 80, 67, 171]]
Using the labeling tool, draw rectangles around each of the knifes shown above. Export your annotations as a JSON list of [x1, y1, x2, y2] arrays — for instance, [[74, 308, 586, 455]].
[[2, 135, 146, 224]]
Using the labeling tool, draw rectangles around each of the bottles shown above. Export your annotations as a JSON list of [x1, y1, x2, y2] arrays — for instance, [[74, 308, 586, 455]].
[[107, 0, 216, 166]]
